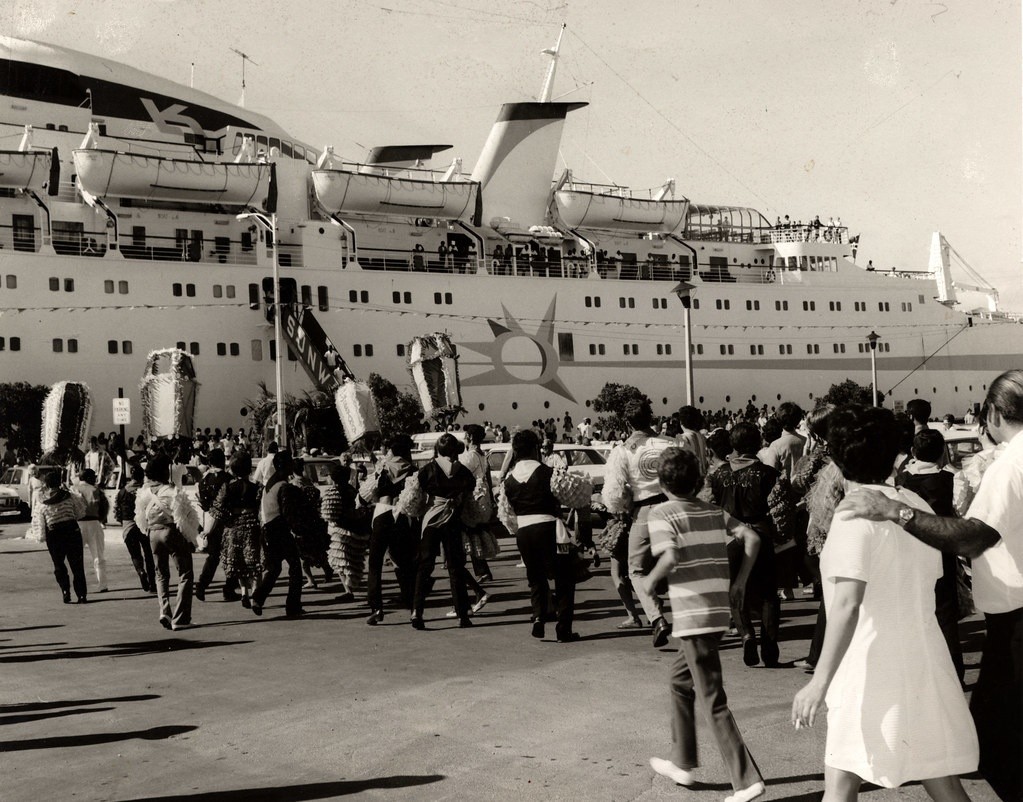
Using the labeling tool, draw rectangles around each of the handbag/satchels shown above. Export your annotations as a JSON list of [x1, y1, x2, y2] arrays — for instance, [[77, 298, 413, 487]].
[[461, 476, 493, 528], [596, 516, 630, 556]]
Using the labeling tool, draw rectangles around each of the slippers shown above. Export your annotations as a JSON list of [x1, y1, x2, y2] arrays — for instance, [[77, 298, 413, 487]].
[[302, 583, 317, 589], [615, 617, 642, 628]]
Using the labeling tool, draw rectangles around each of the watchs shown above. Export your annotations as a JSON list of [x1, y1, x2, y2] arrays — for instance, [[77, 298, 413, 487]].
[[898, 507, 914, 529]]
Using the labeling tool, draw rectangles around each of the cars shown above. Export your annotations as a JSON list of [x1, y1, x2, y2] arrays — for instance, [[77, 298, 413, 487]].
[[102, 445, 614, 533], [0, 467, 31, 522]]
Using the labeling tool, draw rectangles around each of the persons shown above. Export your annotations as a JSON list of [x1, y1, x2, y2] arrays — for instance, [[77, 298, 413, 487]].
[[889, 265, 913, 278], [0, 400, 1023, 642], [867, 260, 875, 273], [766, 265, 777, 283], [324, 345, 354, 385], [410, 237, 558, 278], [839, 371, 1023, 802], [774, 214, 842, 243], [563, 245, 680, 279], [792, 404, 980, 802], [639, 448, 767, 802], [717, 217, 729, 243]]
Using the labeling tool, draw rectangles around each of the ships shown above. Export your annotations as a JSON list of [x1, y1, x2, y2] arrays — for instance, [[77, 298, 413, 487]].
[[0, 21, 1023, 442]]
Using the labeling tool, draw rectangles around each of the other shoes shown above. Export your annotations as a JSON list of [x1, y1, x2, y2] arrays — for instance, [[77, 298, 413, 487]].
[[367, 609, 384, 626], [648, 757, 694, 787], [793, 660, 814, 670], [446, 606, 474, 617], [223, 588, 243, 601], [335, 592, 354, 601], [241, 595, 252, 609], [724, 781, 765, 802], [171, 622, 199, 630], [140, 571, 150, 591], [410, 609, 426, 630], [195, 582, 206, 602], [593, 553, 601, 568], [63, 589, 71, 604], [159, 615, 172, 628], [742, 634, 760, 666], [776, 589, 795, 600], [467, 574, 489, 588], [101, 588, 108, 592], [473, 591, 493, 612], [556, 631, 580, 643], [653, 619, 671, 647], [531, 617, 544, 638], [516, 562, 527, 567], [251, 592, 262, 616], [78, 596, 87, 604], [760, 628, 780, 666]]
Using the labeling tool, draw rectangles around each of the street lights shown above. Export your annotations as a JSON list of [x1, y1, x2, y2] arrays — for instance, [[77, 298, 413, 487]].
[[236, 212, 287, 452], [865, 331, 881, 408], [670, 279, 699, 407]]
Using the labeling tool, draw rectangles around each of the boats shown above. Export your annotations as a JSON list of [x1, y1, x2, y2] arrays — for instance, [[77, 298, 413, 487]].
[[0, 125, 52, 191], [552, 169, 691, 237], [313, 145, 481, 223], [72, 122, 272, 206]]
